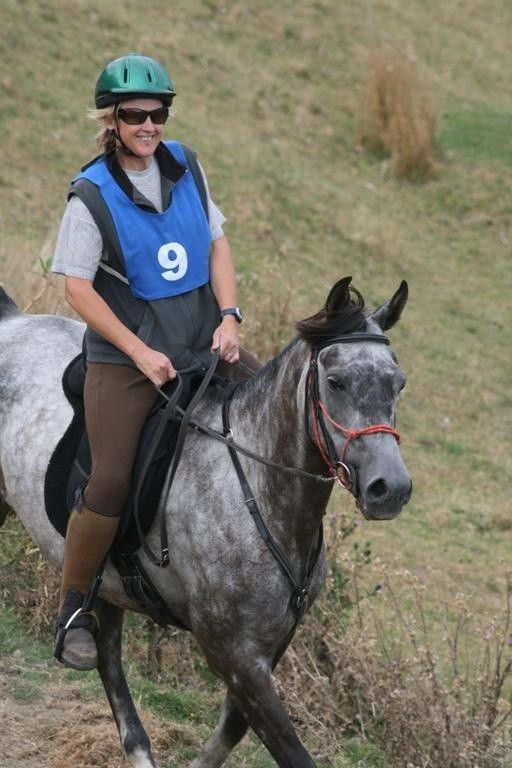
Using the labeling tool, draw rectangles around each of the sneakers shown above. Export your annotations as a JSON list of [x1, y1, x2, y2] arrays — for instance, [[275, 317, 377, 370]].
[[53, 625, 98, 671]]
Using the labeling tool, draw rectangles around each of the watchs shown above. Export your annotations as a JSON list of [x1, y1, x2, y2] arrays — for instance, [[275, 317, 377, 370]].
[[219, 307, 244, 323]]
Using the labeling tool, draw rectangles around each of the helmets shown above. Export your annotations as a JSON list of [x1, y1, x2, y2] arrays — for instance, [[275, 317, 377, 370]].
[[95, 53, 176, 109]]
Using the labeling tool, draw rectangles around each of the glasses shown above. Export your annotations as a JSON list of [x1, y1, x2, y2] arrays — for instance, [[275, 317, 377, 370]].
[[115, 106, 170, 125]]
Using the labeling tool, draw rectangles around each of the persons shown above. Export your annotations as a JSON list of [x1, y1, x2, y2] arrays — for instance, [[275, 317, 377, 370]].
[[51, 50, 271, 672]]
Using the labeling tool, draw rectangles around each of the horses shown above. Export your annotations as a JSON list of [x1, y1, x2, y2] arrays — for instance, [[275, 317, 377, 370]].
[[0, 273, 414, 768]]
